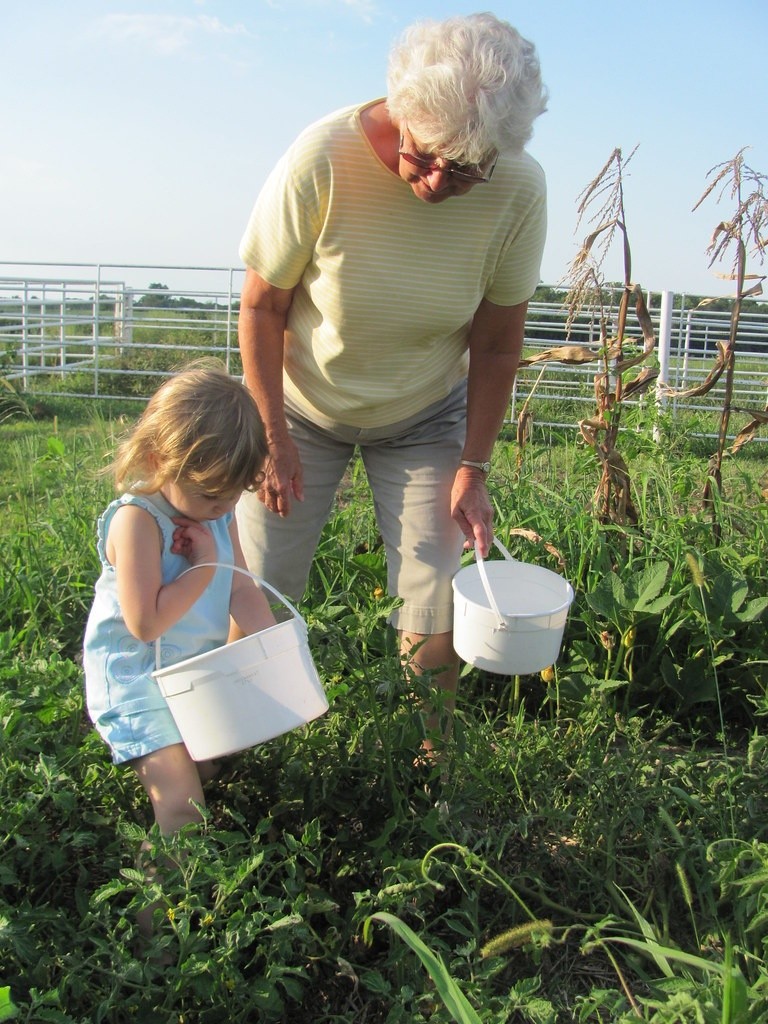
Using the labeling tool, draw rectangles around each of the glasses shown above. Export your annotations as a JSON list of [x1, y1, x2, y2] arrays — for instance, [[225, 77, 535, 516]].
[[398, 122, 499, 184]]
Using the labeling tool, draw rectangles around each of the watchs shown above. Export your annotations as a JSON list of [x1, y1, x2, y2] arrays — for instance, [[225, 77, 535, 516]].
[[461, 461, 492, 473]]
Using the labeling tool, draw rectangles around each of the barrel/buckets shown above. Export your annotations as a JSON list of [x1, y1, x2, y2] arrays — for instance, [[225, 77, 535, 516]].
[[151, 562, 330, 762], [452, 536, 575, 675]]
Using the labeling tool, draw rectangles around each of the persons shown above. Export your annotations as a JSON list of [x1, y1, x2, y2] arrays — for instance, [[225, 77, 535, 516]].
[[81, 370, 278, 936], [232, 11, 548, 784]]
